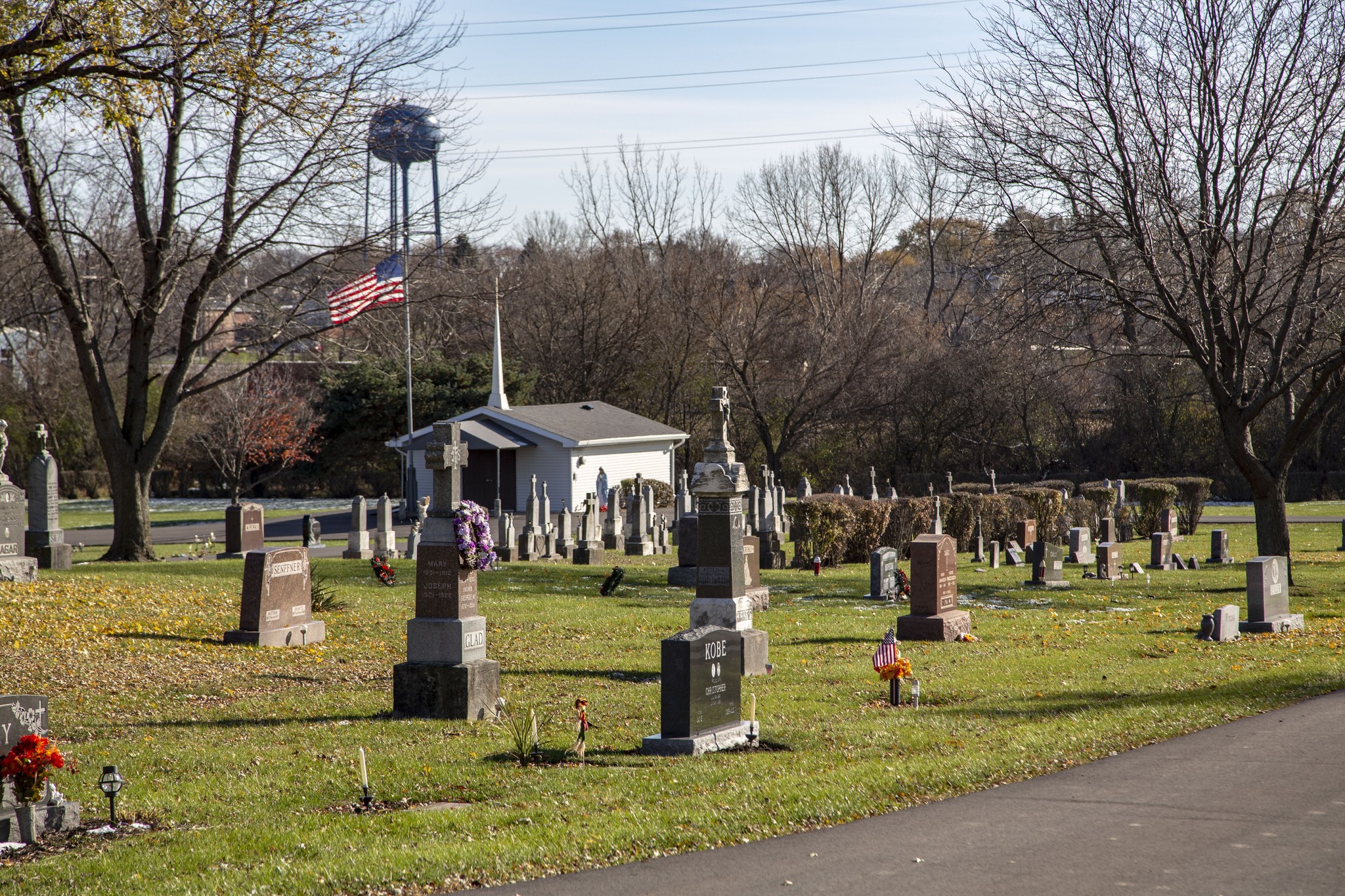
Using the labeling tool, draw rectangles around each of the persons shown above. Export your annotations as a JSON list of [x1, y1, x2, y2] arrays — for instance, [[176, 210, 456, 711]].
[[0, 417, 11, 483], [1099, 564, 1113, 580]]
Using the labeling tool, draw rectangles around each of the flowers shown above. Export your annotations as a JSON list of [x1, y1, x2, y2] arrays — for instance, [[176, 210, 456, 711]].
[[453, 499, 497, 570], [0, 733, 79, 807], [600, 566, 625, 597], [370, 553, 396, 587], [894, 568, 911, 594], [879, 656, 913, 681]]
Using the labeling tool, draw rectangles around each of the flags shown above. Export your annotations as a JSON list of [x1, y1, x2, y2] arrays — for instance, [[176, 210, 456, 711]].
[[870, 628, 897, 673], [326, 247, 406, 324]]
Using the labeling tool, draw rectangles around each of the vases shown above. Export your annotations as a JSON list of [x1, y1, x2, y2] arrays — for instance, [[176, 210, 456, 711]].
[[890, 678, 900, 706], [14, 805, 37, 844]]
